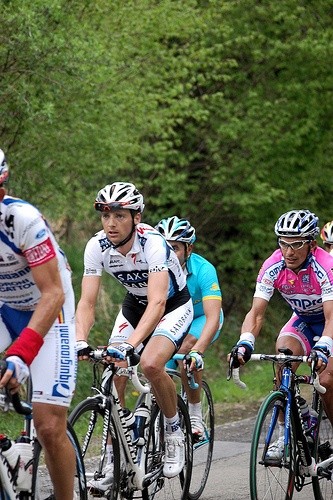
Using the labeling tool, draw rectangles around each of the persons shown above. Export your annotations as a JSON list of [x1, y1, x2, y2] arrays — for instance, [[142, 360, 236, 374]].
[[227, 210, 333, 459], [136, 216, 224, 437], [75, 182, 193, 491], [0, 149, 79, 500], [320, 220, 333, 256]]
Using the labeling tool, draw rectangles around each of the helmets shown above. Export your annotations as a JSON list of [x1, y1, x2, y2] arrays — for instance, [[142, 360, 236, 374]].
[[153, 216, 197, 246], [320, 221, 333, 244], [93, 181, 145, 213], [274, 209, 320, 239], [0, 149, 9, 185]]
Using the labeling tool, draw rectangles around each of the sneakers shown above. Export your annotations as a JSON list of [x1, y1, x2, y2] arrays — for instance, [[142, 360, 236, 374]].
[[266, 436, 285, 461], [189, 415, 204, 437], [85, 463, 114, 490], [163, 426, 186, 478]]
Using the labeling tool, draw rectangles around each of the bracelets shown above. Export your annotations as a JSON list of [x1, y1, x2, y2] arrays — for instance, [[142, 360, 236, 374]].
[[7, 327, 44, 365], [240, 332, 255, 344], [315, 336, 333, 352]]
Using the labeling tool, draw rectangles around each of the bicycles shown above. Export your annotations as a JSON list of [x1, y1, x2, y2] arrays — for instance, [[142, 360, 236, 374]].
[[0, 335, 88, 500], [226, 335, 333, 500], [66, 343, 215, 500]]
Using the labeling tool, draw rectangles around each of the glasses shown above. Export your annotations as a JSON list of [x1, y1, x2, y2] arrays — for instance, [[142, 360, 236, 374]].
[[278, 237, 310, 251]]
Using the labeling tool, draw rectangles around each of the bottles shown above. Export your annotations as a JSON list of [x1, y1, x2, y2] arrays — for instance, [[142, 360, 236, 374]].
[[122, 407, 139, 445], [134, 402, 151, 446], [298, 397, 312, 431], [302, 408, 319, 442], [0, 434, 27, 485], [13, 431, 34, 491]]
